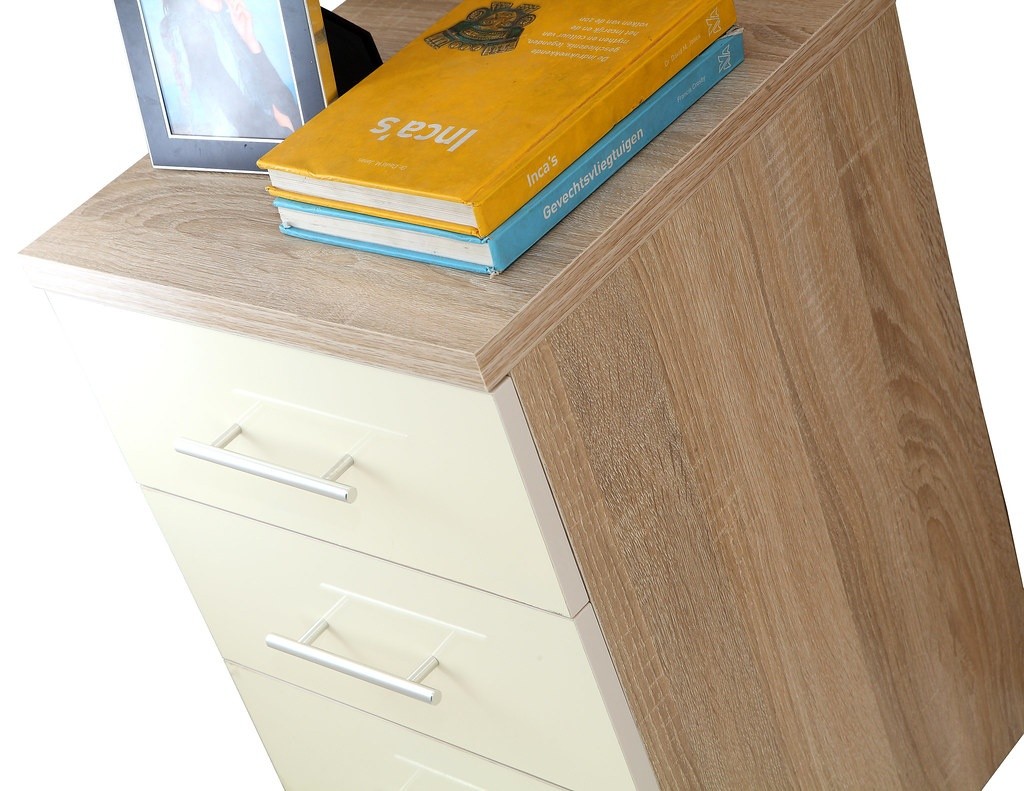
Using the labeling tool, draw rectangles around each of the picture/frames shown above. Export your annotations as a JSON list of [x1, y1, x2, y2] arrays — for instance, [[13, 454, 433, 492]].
[[114, 0, 341, 176]]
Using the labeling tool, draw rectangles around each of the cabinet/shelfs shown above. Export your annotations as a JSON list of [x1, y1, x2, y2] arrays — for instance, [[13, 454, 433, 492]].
[[20, 0, 1023, 791]]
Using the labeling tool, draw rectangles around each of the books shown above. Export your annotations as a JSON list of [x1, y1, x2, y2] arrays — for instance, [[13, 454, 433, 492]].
[[256, 1, 747, 278]]
